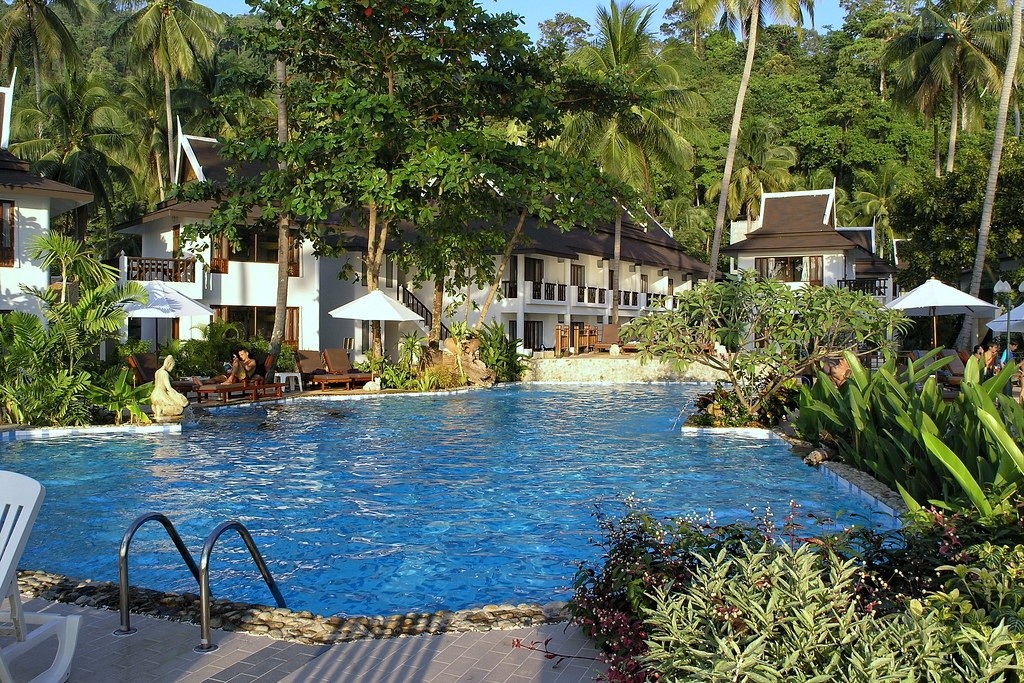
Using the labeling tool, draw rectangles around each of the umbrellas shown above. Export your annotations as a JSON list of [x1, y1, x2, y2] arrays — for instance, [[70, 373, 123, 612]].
[[986, 302, 1024, 334], [878, 278, 999, 390], [327, 289, 425, 381], [113, 277, 215, 370]]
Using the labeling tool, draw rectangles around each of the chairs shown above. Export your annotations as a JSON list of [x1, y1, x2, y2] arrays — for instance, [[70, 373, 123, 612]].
[[128, 353, 209, 403], [0, 467, 83, 683], [193, 352, 286, 404], [131, 261, 190, 282], [910, 349, 1024, 400], [593, 324, 714, 355], [292, 347, 373, 391]]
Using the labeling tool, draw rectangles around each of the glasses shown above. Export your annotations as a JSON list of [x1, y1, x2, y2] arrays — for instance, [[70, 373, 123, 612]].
[[978, 352, 982, 356]]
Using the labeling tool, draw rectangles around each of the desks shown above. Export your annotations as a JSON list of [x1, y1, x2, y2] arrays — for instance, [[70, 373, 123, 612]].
[[179, 377, 210, 381], [273, 372, 304, 393]]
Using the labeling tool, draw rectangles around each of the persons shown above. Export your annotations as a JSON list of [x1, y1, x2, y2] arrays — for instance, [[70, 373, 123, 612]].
[[964, 339, 1024, 405], [192, 348, 256, 386], [150, 354, 189, 417]]
